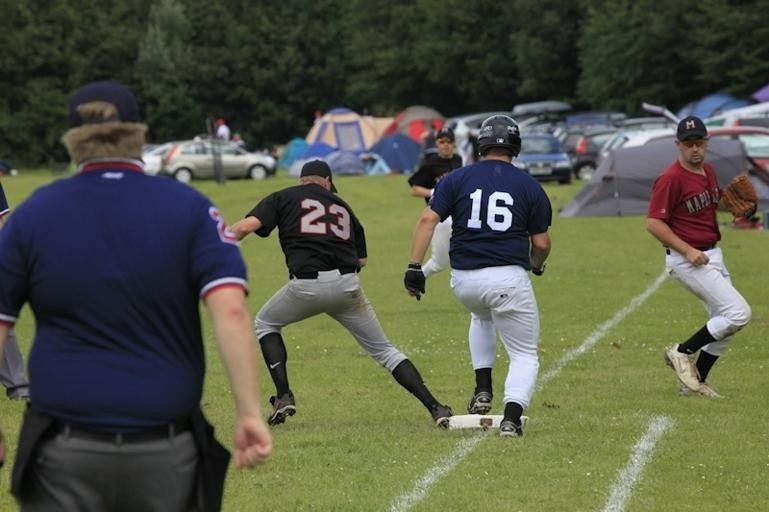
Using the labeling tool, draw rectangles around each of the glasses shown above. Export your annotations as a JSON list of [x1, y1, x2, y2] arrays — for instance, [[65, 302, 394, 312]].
[[678, 139, 709, 148], [437, 139, 453, 143]]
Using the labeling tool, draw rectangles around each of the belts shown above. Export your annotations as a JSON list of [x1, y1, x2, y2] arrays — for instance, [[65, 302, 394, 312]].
[[44, 418, 193, 441], [667, 245, 719, 257], [295, 268, 358, 279]]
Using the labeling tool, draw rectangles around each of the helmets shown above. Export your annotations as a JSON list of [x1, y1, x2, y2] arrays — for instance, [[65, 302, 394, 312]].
[[479, 116, 522, 157]]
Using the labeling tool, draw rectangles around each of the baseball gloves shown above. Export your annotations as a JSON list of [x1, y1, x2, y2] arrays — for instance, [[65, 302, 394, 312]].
[[723, 174, 758, 219]]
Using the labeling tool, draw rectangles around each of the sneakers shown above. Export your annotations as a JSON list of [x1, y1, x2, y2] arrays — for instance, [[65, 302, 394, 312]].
[[500, 420, 525, 440], [664, 343, 703, 392], [433, 405, 453, 429], [268, 395, 297, 426], [465, 391, 491, 414], [680, 380, 724, 399]]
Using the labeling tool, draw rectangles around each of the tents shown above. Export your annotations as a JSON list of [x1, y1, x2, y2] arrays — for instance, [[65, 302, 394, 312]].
[[277, 138, 311, 169], [304, 107, 378, 154], [289, 157, 333, 176], [324, 148, 365, 175], [366, 132, 424, 174], [558, 127, 750, 217], [381, 106, 446, 145], [299, 141, 335, 159]]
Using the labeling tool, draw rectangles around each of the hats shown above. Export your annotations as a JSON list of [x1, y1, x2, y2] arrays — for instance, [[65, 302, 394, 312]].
[[69, 81, 142, 126], [433, 125, 456, 139], [301, 161, 340, 196], [677, 116, 708, 141]]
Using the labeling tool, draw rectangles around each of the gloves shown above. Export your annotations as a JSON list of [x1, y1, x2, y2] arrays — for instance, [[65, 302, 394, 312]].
[[404, 263, 426, 301], [532, 265, 546, 277]]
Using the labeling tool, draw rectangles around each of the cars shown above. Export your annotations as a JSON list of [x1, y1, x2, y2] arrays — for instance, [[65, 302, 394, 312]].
[[599, 130, 677, 156], [615, 117, 673, 130], [646, 127, 769, 173], [703, 114, 769, 126], [563, 131, 619, 179], [146, 141, 278, 181], [510, 101, 625, 130], [512, 132, 572, 183], [448, 113, 510, 134]]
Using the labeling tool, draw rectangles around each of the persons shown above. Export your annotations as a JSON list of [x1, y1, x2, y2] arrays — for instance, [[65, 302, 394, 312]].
[[217, 118, 230, 141], [447, 120, 458, 151], [647, 116, 758, 401], [0, 182, 30, 399], [421, 123, 438, 148], [231, 133, 244, 146], [0, 79, 272, 512], [404, 115, 552, 438], [229, 160, 452, 430], [407, 127, 463, 296]]
